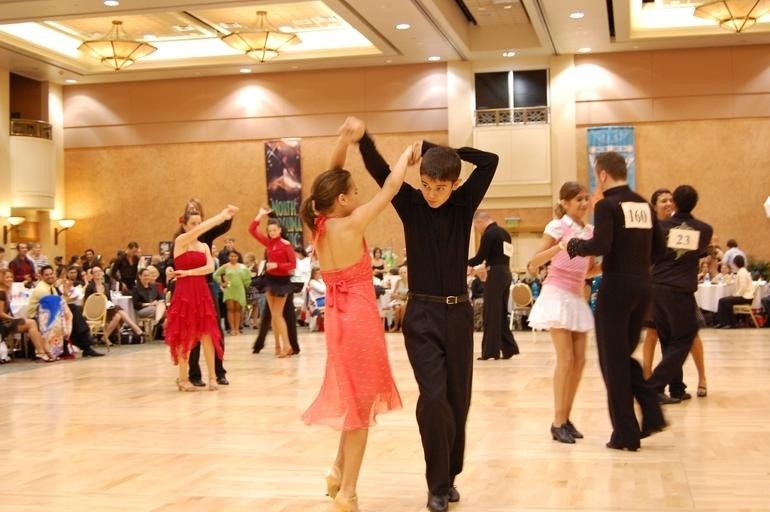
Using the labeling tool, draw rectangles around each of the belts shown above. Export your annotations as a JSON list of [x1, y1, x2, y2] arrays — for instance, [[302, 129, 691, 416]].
[[407, 291, 471, 305]]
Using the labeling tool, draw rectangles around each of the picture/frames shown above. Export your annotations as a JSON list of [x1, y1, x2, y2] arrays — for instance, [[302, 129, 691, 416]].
[[159, 241, 171, 255]]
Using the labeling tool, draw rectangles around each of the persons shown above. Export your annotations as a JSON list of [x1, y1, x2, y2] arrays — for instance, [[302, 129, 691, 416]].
[[164, 197, 239, 386], [347, 115, 500, 512], [646, 183, 715, 404], [290, 247, 326, 332], [520, 265, 547, 331], [527, 180, 594, 444], [584, 275, 601, 309], [642, 187, 708, 398], [468, 264, 489, 332], [252, 209, 301, 356], [0, 242, 167, 362], [563, 150, 669, 453], [295, 113, 418, 512], [371, 247, 409, 333], [211, 240, 263, 336], [467, 210, 520, 362], [697, 239, 754, 329], [160, 204, 235, 392], [247, 204, 305, 359]]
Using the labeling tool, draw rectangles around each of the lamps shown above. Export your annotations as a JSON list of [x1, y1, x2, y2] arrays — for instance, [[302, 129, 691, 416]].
[[221, 11, 302, 63], [78, 20, 157, 71], [54, 217, 75, 244], [3, 217, 26, 243], [693, 0, 770, 35]]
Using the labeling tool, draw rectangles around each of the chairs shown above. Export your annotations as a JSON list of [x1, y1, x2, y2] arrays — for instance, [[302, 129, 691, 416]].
[[733, 280, 762, 328], [82, 292, 110, 353], [510, 282, 535, 332]]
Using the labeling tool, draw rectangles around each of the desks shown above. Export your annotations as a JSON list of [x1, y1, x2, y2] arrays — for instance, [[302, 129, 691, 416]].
[[698, 281, 770, 327], [72, 295, 134, 344]]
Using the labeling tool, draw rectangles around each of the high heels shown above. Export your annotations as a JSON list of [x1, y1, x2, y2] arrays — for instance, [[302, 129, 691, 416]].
[[34, 349, 60, 362], [175, 376, 200, 391], [100, 338, 114, 346], [549, 420, 584, 443], [696, 386, 708, 396], [134, 331, 148, 339], [207, 380, 219, 391], [277, 345, 293, 358]]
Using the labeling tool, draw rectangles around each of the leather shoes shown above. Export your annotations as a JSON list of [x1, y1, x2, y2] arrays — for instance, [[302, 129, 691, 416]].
[[477, 350, 520, 361], [322, 473, 362, 512], [81, 348, 106, 357], [216, 375, 229, 386], [428, 484, 460, 510], [189, 377, 206, 386], [652, 392, 692, 404], [605, 439, 641, 451]]
